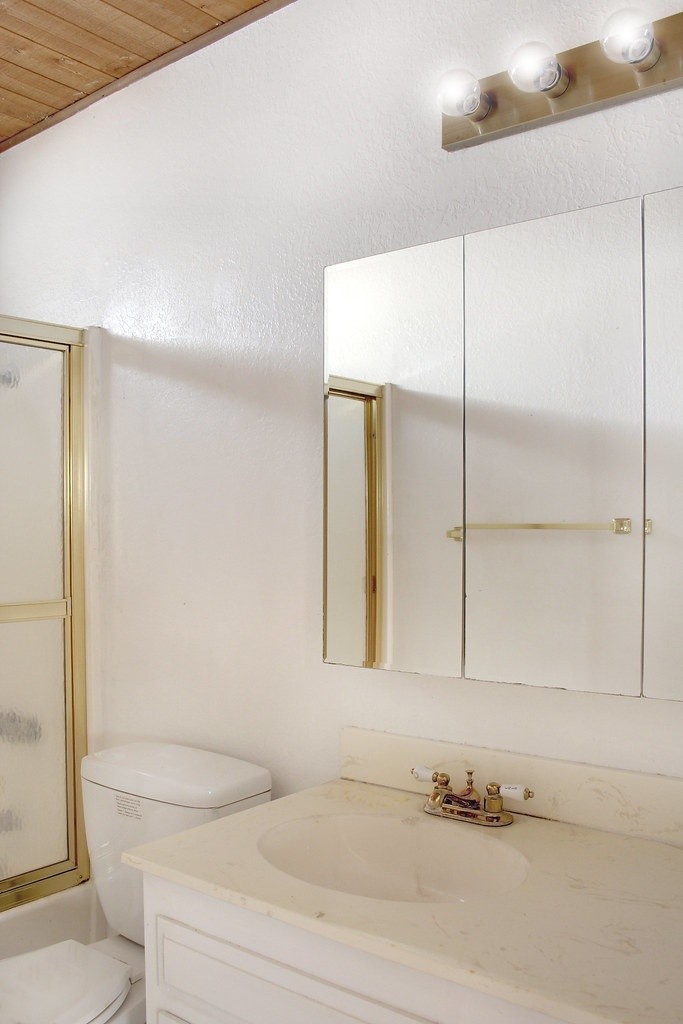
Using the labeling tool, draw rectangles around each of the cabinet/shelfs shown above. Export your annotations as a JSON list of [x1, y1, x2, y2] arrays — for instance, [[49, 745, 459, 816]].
[[155, 912, 441, 1023]]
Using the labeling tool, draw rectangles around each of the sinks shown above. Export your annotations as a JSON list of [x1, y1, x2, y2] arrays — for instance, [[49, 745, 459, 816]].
[[256, 814, 529, 904]]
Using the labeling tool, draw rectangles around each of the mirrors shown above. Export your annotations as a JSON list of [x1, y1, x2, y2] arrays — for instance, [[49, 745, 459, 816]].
[[322, 187, 683, 702]]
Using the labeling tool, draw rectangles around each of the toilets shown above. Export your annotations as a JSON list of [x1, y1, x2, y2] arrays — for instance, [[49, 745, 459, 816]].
[[0, 741, 274, 1024]]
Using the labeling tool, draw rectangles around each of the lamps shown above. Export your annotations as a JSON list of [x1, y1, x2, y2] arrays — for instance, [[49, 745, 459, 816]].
[[437, 8, 683, 153]]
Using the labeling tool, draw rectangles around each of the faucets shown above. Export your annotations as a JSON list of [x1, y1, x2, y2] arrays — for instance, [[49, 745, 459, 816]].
[[426, 769, 481, 808]]
